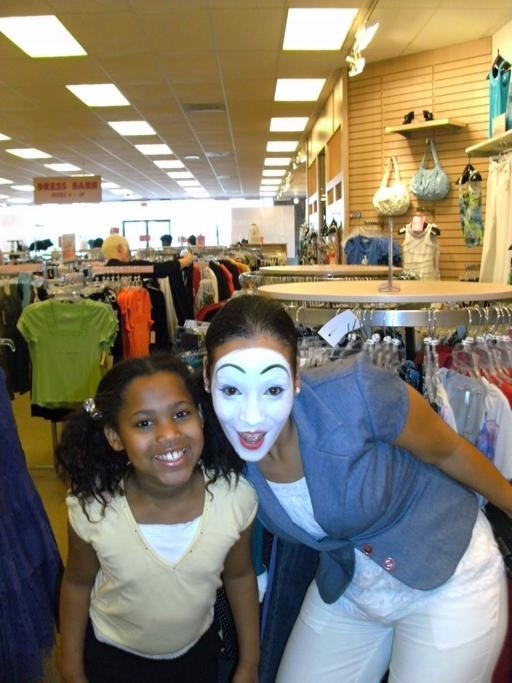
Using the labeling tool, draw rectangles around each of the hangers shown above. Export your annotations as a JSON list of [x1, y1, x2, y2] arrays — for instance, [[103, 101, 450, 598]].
[[25, 266, 147, 318], [183, 301, 420, 384], [431, 304, 512, 407]]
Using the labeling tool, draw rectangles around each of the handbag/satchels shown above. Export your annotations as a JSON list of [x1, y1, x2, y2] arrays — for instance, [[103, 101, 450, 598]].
[[411, 168, 450, 200], [373, 184, 411, 216]]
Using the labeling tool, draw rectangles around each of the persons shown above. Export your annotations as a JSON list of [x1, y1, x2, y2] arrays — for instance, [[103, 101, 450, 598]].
[[57, 353, 260, 683], [202, 295, 512, 683], [102, 234, 194, 278], [160, 235, 172, 246]]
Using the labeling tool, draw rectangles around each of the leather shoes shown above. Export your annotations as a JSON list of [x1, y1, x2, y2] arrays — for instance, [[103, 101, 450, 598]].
[[403, 111, 433, 124]]
